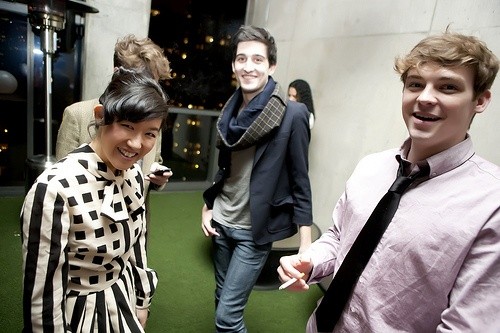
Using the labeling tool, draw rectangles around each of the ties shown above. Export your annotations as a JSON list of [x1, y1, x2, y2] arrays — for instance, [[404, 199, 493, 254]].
[[314, 157, 433, 333]]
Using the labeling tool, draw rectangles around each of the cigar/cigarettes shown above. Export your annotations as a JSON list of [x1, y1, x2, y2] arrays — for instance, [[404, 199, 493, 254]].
[[278, 273, 305, 292]]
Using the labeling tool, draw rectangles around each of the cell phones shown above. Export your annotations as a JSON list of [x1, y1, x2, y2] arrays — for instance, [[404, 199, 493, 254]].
[[147, 168, 171, 177]]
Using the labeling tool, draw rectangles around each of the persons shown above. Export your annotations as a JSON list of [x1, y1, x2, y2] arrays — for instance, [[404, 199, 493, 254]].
[[275, 21, 500, 333], [200, 23, 314, 333], [17, 66, 172, 333], [55, 32, 173, 259], [286, 79, 316, 131]]
